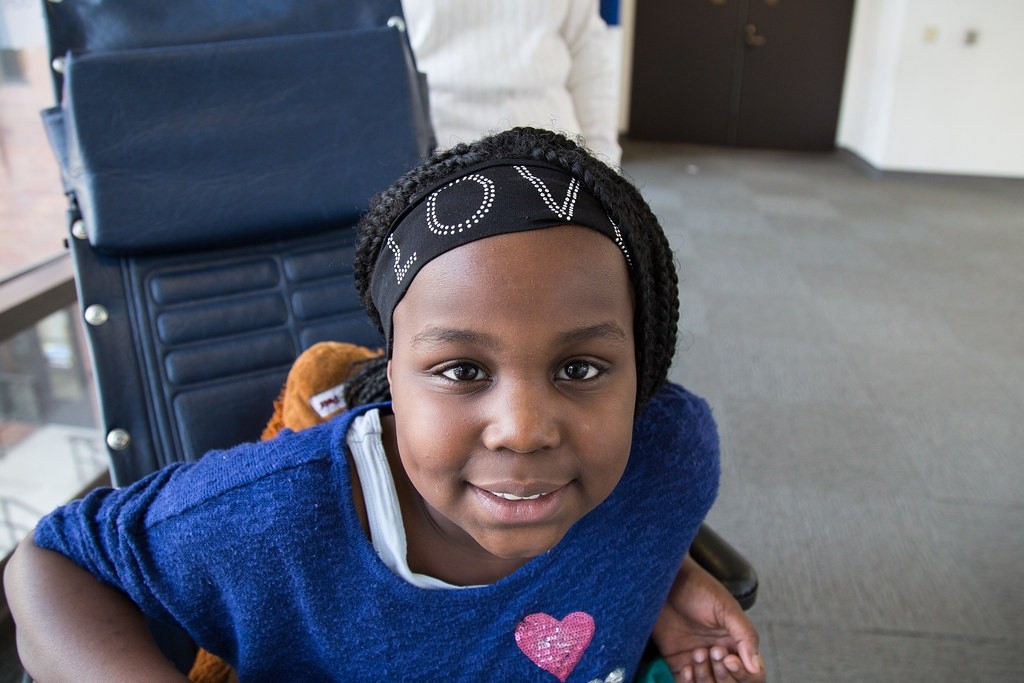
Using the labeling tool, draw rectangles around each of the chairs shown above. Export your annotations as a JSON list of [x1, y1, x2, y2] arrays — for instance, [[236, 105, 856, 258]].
[[41, 0, 761, 609]]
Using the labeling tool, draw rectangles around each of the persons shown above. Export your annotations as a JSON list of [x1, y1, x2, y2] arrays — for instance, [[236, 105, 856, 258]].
[[399, 0, 623, 172], [3, 123, 766, 683]]
